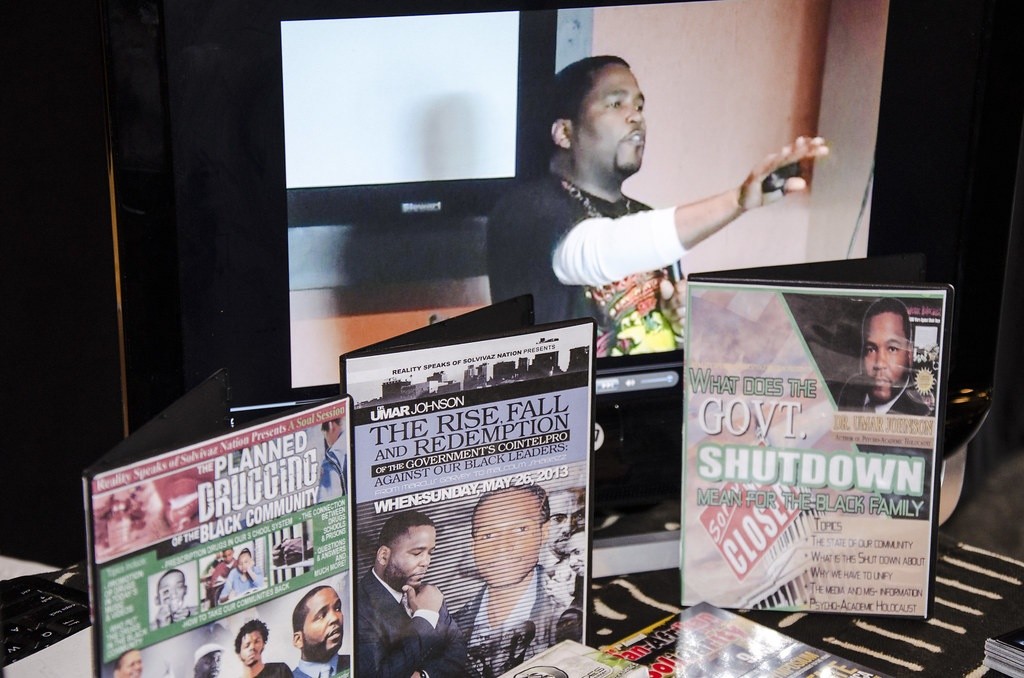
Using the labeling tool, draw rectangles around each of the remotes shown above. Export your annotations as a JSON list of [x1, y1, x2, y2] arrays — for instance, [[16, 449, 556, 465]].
[[763, 162, 803, 193]]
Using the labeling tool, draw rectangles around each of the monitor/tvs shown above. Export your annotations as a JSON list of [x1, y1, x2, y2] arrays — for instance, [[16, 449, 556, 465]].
[[279, 7, 596, 291], [162, 0, 995, 516]]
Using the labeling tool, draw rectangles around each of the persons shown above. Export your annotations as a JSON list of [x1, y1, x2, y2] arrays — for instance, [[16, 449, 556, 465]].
[[316, 418, 348, 504], [112, 586, 349, 678], [485, 56, 830, 358], [838, 299, 935, 420], [358, 484, 585, 678], [153, 537, 314, 627]]
